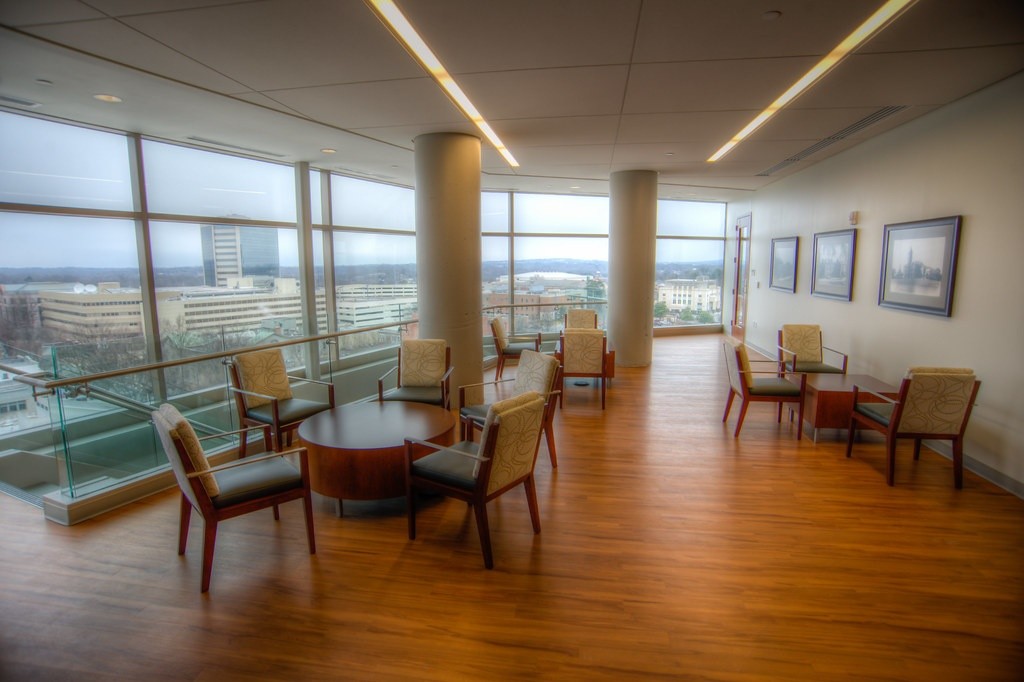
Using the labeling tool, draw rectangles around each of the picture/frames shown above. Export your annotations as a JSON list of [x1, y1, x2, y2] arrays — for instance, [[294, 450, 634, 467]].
[[768, 236, 799, 294], [877, 215, 962, 318], [809, 228, 857, 302]]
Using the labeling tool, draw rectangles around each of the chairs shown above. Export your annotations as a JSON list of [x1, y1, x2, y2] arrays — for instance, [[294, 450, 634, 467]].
[[490, 315, 542, 385], [221, 348, 335, 461], [152, 403, 315, 595], [558, 332, 607, 410], [564, 308, 598, 329], [778, 324, 848, 422], [403, 391, 547, 570], [458, 349, 564, 468], [846, 366, 982, 491], [377, 338, 454, 411], [722, 339, 807, 440]]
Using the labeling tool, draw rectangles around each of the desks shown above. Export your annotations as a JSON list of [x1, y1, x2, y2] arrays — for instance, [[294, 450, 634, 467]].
[[555, 340, 615, 383], [297, 401, 458, 519], [788, 373, 898, 446]]
